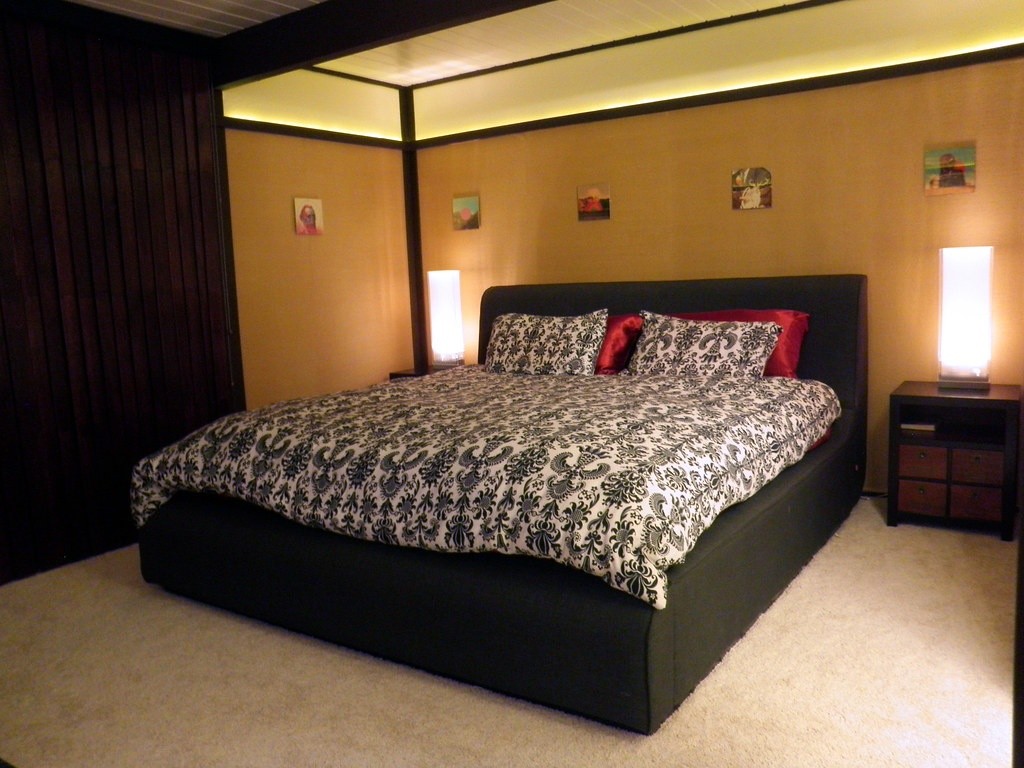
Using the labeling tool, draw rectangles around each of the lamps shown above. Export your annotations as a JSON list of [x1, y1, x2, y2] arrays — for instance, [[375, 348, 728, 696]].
[[428, 270, 462, 370], [938, 245, 992, 391]]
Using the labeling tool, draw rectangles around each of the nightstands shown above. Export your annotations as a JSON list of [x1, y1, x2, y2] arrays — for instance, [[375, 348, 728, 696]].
[[887, 380, 1021, 542], [389, 367, 442, 382]]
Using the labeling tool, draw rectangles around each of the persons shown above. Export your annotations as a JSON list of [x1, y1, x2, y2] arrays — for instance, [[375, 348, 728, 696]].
[[298, 204, 321, 235], [938, 153, 966, 188]]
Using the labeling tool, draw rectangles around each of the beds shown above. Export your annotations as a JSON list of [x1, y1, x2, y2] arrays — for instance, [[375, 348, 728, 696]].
[[130, 275, 866, 737]]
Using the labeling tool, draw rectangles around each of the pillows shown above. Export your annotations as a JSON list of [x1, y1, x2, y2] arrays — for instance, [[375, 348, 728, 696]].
[[483, 307, 608, 374], [619, 309, 784, 380]]
